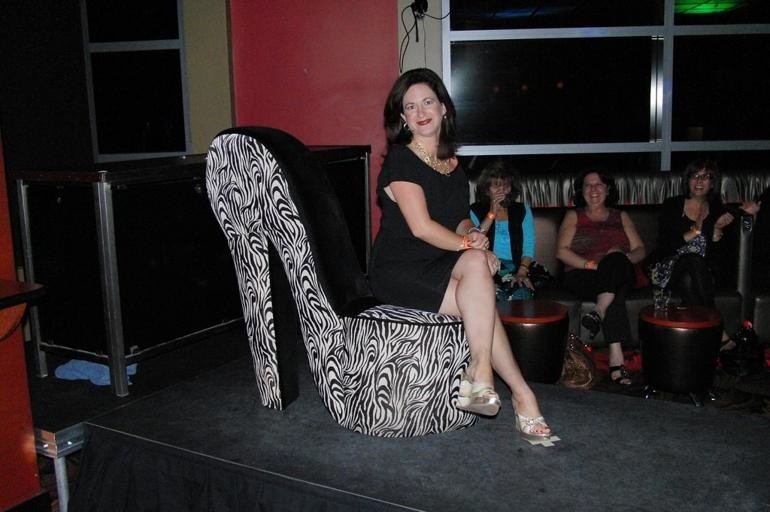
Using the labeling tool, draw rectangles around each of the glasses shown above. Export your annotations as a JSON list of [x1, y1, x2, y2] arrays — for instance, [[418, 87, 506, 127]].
[[690, 172, 713, 180]]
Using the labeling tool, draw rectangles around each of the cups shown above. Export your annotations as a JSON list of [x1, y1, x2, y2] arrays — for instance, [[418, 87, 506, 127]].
[[499, 198, 512, 209], [655, 298, 666, 310]]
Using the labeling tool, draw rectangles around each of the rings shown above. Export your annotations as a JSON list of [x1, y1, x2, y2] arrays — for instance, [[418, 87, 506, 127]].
[[484, 245, 488, 251], [525, 277, 530, 282]]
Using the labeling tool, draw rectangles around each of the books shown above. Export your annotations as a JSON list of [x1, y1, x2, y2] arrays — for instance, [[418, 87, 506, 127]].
[[722, 175, 770, 203], [515, 177, 575, 207], [469, 179, 476, 203], [614, 176, 683, 204]]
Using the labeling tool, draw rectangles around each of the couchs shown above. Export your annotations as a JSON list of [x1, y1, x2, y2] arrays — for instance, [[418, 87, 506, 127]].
[[530, 202, 770, 346]]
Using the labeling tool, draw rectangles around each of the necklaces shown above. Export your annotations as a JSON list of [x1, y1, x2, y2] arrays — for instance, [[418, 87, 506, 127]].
[[408, 137, 452, 179]]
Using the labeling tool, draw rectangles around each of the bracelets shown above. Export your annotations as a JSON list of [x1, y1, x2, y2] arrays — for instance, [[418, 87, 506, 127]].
[[463, 234, 472, 250], [519, 264, 529, 272], [468, 226, 480, 233], [712, 232, 724, 239], [625, 251, 632, 261], [689, 225, 702, 235], [487, 212, 495, 220], [584, 258, 597, 270]]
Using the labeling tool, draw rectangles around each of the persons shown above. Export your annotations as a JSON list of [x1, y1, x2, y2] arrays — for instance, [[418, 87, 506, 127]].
[[728, 179, 770, 353], [364, 66, 562, 453], [653, 155, 745, 379], [552, 160, 648, 392], [470, 158, 537, 303]]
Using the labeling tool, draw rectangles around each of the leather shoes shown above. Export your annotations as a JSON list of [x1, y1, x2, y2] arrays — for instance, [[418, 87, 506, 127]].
[[719, 338, 737, 352]]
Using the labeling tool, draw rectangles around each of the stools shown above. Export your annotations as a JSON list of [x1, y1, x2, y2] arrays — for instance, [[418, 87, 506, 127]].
[[636, 302, 725, 400], [496, 298, 569, 385]]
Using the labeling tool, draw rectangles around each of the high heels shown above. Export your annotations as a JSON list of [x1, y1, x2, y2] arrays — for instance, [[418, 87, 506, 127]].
[[456, 371, 501, 416], [608, 365, 632, 385], [511, 393, 552, 440], [582, 310, 602, 340]]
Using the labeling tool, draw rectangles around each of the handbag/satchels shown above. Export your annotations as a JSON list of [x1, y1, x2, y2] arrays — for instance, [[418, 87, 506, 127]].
[[559, 333, 597, 391], [642, 249, 674, 288], [527, 261, 557, 290], [720, 328, 764, 376]]
